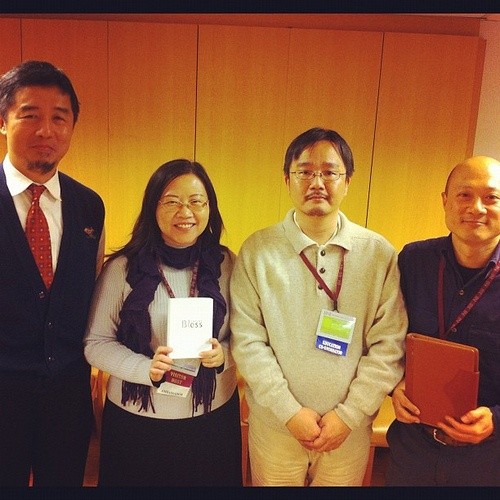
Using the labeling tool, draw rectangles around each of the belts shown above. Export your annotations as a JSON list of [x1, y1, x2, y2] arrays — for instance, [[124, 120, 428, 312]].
[[425, 423, 468, 448]]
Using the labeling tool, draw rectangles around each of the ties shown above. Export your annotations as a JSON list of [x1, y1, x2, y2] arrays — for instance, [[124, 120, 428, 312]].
[[25, 185, 54, 289]]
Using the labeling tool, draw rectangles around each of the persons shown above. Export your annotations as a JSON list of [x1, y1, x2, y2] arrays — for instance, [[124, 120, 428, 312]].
[[396, 156, 500, 486], [0, 59, 107, 487], [82, 159, 244, 487], [229, 128, 410, 487]]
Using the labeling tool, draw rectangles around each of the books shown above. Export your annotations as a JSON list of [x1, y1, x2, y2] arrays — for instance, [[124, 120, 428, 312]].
[[405, 332, 480, 433]]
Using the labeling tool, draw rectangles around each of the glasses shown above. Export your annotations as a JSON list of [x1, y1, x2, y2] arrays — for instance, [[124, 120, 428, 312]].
[[289, 169, 348, 182], [155, 196, 210, 212]]
